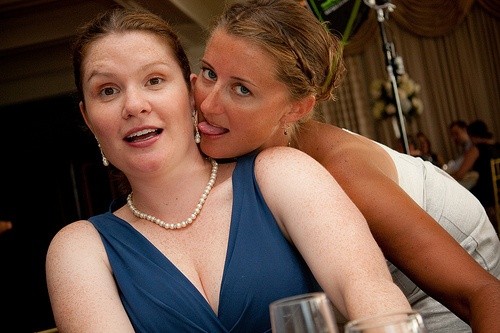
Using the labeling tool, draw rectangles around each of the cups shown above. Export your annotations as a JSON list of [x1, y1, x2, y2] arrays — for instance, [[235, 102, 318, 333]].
[[345, 310, 426, 333], [269, 292, 338, 333]]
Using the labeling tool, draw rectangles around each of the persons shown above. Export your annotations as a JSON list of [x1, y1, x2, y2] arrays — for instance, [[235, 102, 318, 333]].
[[416, 120, 500, 204], [194, 0, 500, 333], [45, 6, 419, 333]]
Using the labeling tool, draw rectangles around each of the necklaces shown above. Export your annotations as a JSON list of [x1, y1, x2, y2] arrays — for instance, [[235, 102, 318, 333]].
[[112, 158, 220, 229]]
